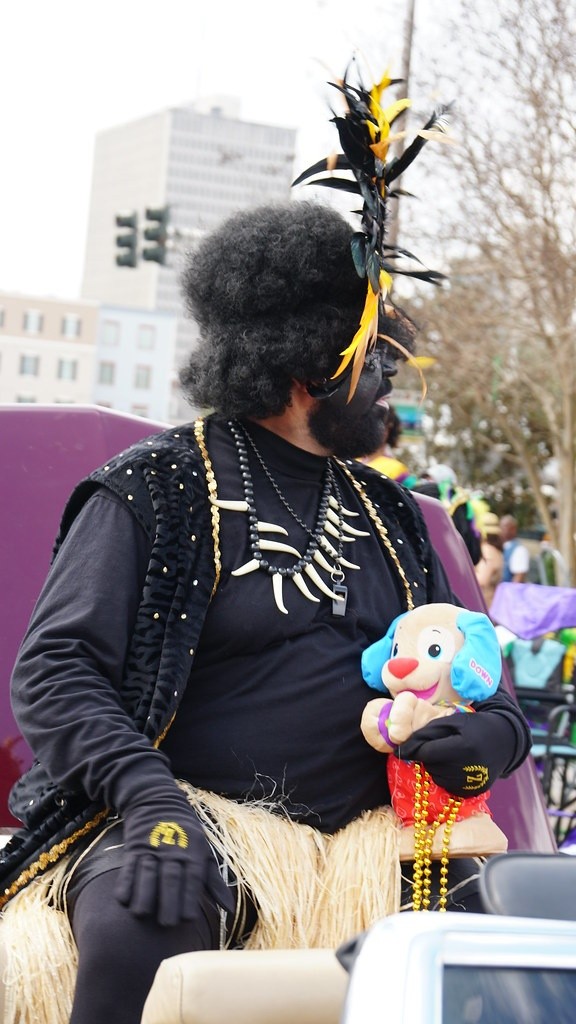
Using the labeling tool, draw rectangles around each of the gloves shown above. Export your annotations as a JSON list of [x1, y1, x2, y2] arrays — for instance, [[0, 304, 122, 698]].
[[115, 785, 237, 931], [396, 711, 518, 798]]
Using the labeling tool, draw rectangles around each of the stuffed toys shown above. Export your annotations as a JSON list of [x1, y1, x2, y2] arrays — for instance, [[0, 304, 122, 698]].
[[360, 603, 503, 826]]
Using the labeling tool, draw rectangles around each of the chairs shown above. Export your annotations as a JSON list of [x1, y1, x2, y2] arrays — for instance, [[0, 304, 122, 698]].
[[1, 403, 576, 920]]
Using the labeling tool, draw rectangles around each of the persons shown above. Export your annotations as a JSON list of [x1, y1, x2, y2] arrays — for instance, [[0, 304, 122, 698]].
[[9, 200, 535, 1024], [356, 396, 502, 568], [532, 525, 569, 588], [497, 515, 531, 583]]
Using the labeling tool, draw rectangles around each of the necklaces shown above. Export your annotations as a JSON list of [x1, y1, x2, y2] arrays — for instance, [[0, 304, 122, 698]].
[[228, 413, 348, 616], [411, 762, 461, 912]]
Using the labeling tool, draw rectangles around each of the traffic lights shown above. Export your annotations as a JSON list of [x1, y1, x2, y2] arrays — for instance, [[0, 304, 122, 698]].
[[115, 212, 136, 268], [143, 205, 167, 266]]
